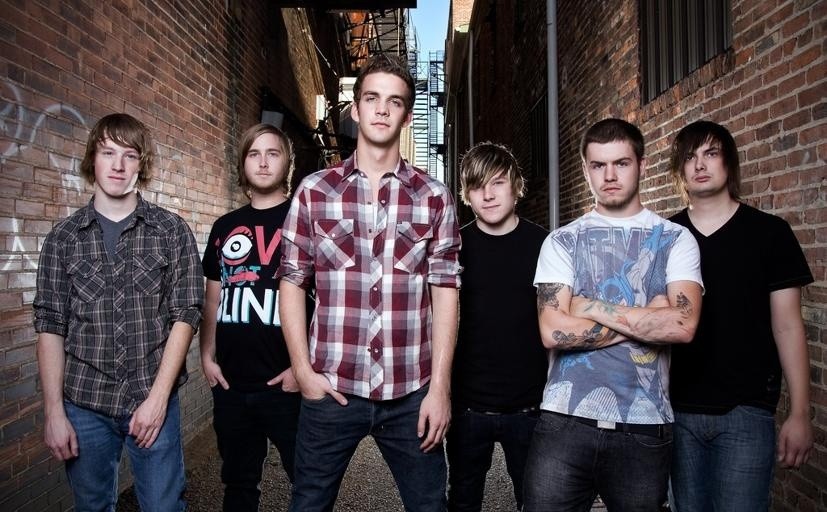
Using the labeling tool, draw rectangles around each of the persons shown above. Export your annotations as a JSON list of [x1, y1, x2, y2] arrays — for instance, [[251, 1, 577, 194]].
[[668, 121, 815, 512], [200, 122, 317, 512], [522, 118, 705, 512], [32, 113, 204, 511], [445, 140, 551, 512], [276, 54, 465, 512]]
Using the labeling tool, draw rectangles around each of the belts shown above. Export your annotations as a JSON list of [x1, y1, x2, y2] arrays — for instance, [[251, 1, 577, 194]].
[[540, 409, 667, 440]]
